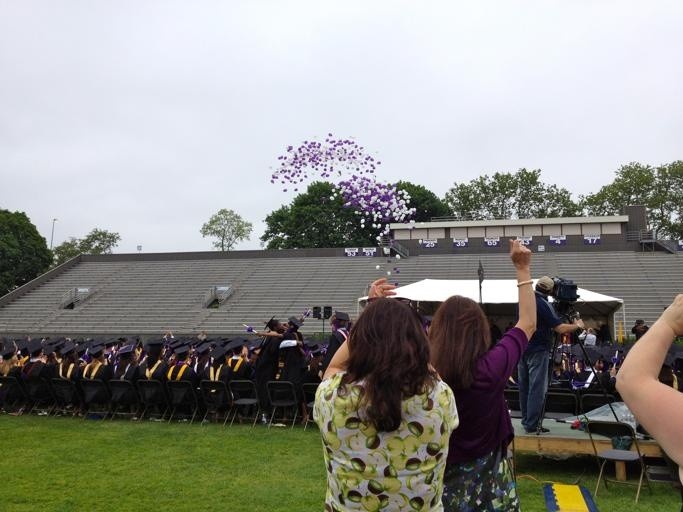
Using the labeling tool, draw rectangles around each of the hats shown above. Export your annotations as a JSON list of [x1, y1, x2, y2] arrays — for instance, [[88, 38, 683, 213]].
[[288, 316, 304, 328], [553, 344, 634, 367], [537, 275, 554, 291], [663, 342, 683, 368], [264, 315, 278, 331], [336, 312, 350, 321]]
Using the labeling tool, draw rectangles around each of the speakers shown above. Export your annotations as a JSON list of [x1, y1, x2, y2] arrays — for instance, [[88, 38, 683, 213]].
[[313, 307, 321, 318], [323, 306, 332, 318]]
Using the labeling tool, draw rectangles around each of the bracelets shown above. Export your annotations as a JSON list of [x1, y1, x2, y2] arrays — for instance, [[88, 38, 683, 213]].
[[517, 280, 533, 288]]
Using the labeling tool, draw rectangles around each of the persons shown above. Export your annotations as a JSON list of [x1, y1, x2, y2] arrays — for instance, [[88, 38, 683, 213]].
[[631, 320, 649, 341], [517, 275, 585, 433], [425, 237, 538, 509], [312, 278, 460, 512], [1, 311, 354, 426], [613, 292, 682, 494], [488, 317, 501, 349], [554, 325, 631, 394], [658, 337, 682, 391]]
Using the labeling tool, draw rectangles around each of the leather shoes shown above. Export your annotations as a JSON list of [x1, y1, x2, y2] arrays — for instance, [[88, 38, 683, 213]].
[[525, 427, 550, 433]]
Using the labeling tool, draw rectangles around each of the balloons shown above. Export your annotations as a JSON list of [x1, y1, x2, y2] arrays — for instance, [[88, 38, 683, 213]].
[[270, 131, 419, 287]]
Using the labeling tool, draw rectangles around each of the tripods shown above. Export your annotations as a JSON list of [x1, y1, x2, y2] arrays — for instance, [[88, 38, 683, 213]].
[[536, 307, 620, 436]]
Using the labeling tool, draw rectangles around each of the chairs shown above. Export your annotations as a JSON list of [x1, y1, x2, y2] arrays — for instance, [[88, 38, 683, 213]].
[[586, 421, 653, 503]]
